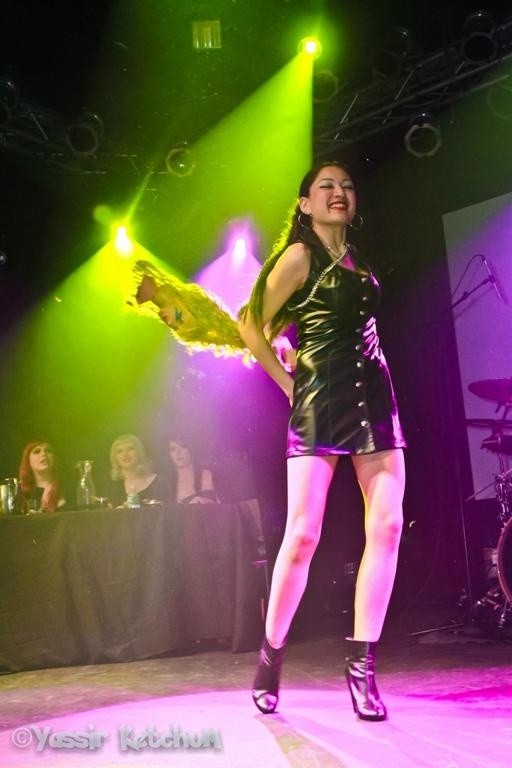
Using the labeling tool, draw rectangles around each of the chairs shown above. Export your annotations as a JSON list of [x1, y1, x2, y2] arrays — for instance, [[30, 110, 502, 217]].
[[234, 498, 270, 604]]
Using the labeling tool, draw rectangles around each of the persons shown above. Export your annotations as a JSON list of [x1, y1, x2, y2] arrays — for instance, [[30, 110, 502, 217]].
[[153, 429, 221, 505], [235, 160, 410, 723], [94, 434, 161, 508], [15, 437, 77, 514]]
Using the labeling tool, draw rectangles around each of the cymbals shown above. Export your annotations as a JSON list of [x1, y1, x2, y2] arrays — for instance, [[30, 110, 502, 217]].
[[459, 418, 512, 431], [467, 377, 512, 409]]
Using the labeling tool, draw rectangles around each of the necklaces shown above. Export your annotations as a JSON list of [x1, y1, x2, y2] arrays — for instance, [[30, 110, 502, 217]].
[[286, 243, 352, 311]]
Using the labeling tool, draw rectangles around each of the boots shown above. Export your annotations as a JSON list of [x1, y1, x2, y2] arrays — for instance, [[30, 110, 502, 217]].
[[252, 631, 289, 714], [344, 637, 386, 721]]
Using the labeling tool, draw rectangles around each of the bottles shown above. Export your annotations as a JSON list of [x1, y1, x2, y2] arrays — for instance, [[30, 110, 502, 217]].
[[76, 459, 97, 511], [128, 487, 141, 510]]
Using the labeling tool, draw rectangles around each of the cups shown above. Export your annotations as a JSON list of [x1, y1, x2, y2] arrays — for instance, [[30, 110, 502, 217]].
[[1, 479, 18, 514]]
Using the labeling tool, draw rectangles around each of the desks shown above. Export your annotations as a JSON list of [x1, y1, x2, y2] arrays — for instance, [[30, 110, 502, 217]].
[[0, 500, 257, 674]]
